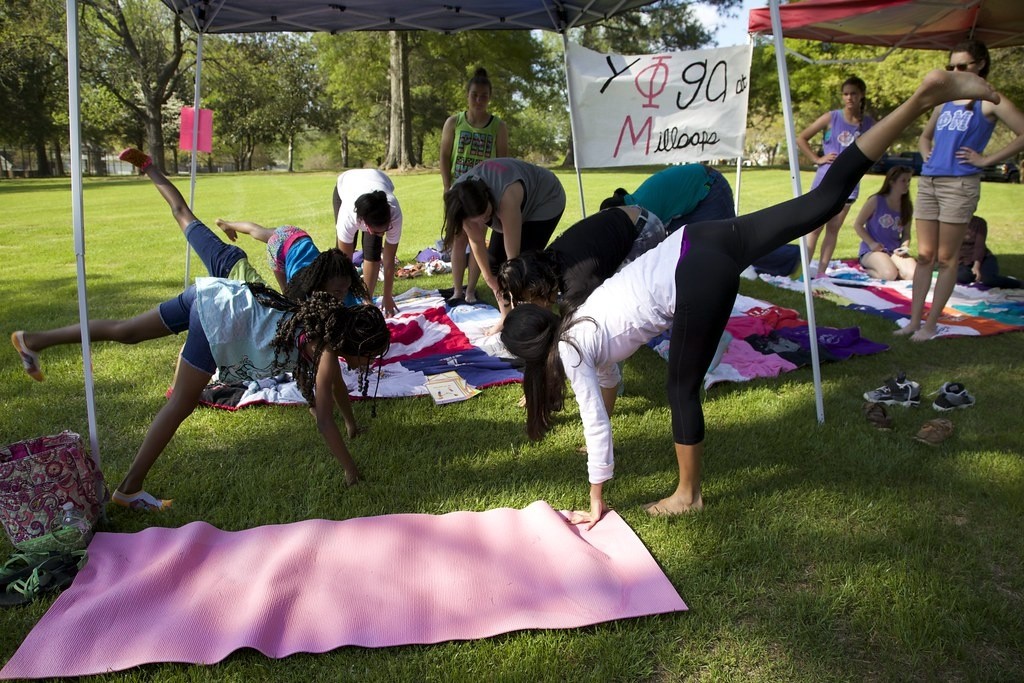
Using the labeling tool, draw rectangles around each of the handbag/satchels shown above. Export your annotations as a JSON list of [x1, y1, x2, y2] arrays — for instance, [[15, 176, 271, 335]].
[[15, 517, 87, 551], [0, 432, 110, 546]]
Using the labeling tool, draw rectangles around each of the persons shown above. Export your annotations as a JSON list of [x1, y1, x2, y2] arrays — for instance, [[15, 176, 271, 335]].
[[598, 160, 800, 280], [853, 165, 918, 281], [118, 148, 274, 289], [215, 216, 367, 300], [795, 75, 876, 279], [439, 157, 566, 336], [439, 68, 507, 302], [955, 216, 1024, 290], [501, 68, 1000, 530], [10, 276, 391, 511], [497, 202, 666, 374], [330, 169, 404, 316], [888, 40, 1024, 341]]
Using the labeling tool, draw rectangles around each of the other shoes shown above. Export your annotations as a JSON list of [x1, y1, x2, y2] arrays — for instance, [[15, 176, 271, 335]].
[[0, 550, 88, 605], [862, 402, 895, 433], [912, 419, 955, 446]]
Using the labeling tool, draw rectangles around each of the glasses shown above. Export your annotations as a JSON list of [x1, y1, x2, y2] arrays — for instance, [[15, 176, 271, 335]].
[[366, 220, 393, 235], [945, 60, 976, 71]]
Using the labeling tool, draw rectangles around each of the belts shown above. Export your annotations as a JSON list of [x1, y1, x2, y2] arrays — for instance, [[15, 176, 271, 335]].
[[635, 208, 648, 240], [704, 168, 718, 192]]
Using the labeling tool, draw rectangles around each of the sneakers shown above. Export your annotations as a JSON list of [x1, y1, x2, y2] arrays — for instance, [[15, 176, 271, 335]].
[[933, 381, 975, 411], [863, 372, 921, 408]]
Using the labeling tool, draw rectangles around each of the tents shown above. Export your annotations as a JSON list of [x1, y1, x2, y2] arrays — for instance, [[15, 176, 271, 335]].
[[734, 0, 1023, 217], [65, 0, 825, 476]]
